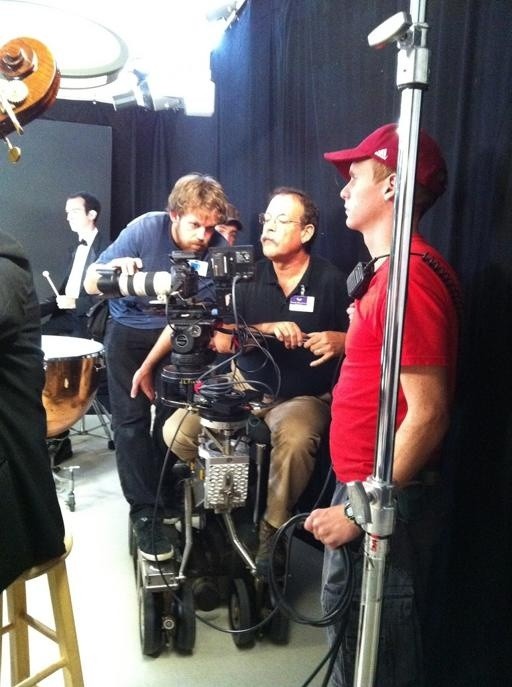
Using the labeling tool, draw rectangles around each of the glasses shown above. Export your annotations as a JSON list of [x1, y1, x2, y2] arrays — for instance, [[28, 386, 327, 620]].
[[258, 211, 300, 225]]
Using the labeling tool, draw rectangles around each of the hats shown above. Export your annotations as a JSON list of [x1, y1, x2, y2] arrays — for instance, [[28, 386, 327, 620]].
[[223, 203, 242, 231], [323, 124, 448, 196]]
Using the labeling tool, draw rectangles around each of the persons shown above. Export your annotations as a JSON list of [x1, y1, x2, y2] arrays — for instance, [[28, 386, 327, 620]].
[[161, 182, 353, 576], [321, 124, 460, 687], [39, 191, 113, 462], [83, 174, 236, 565], [217, 205, 245, 248], [0, 226, 65, 594]]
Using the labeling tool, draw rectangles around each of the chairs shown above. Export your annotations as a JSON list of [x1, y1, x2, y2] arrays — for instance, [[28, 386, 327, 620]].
[[47, 298, 115, 473]]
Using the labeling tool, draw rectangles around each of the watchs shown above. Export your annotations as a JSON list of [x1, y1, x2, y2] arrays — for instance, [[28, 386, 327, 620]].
[[344, 497, 364, 534]]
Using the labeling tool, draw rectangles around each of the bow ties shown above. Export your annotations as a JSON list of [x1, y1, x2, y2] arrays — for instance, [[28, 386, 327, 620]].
[[74, 239, 87, 246]]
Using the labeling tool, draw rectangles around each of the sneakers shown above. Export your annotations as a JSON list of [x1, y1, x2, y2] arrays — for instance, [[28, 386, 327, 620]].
[[132, 515, 174, 562], [255, 520, 287, 576]]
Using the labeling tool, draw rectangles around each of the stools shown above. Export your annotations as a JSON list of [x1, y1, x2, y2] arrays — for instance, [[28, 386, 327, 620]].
[[0, 534, 84, 687]]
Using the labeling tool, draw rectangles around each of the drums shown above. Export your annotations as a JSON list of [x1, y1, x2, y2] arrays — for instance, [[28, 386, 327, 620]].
[[40, 334, 107, 440]]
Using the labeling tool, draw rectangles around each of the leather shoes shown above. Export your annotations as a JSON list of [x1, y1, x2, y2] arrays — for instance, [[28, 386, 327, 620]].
[[49, 438, 71, 465]]
